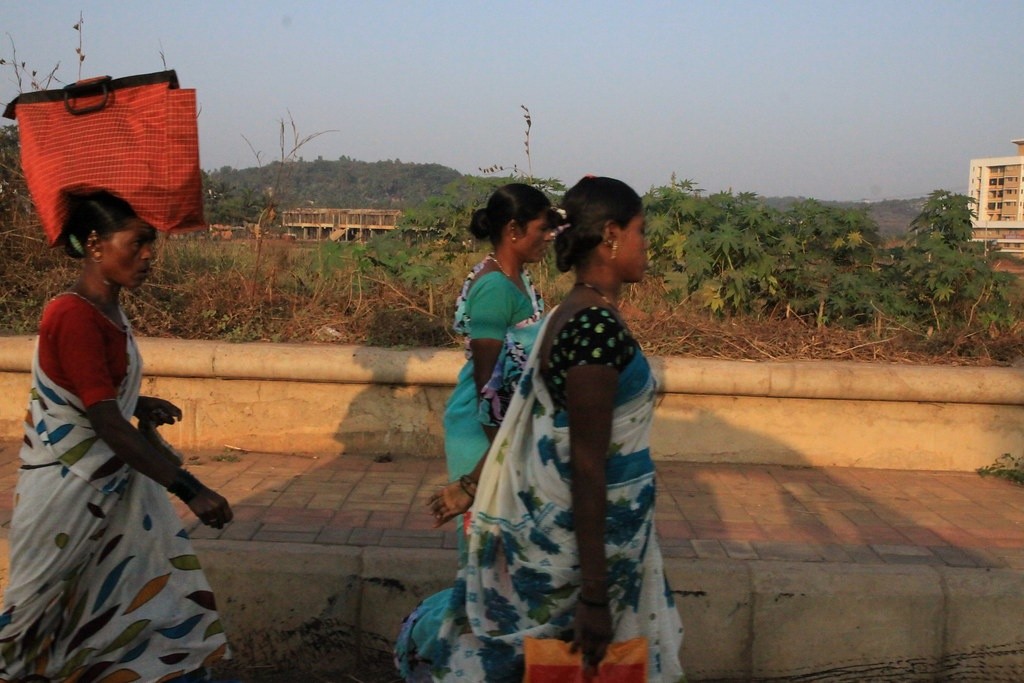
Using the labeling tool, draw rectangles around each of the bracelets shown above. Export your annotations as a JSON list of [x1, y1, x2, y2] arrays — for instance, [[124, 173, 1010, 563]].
[[576, 574, 612, 607], [166, 467, 203, 502], [458, 474, 477, 502]]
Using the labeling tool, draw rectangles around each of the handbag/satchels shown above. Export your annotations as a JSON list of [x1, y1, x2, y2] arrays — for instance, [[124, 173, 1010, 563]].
[[524, 636, 650, 683], [3, 70, 212, 249]]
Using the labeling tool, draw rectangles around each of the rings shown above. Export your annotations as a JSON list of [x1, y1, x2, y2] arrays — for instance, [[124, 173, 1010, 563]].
[[210, 518, 216, 522]]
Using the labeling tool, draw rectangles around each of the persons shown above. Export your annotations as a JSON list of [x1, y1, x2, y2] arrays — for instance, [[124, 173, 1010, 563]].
[[443, 182, 557, 559], [4, 189, 233, 683], [426, 174, 687, 683]]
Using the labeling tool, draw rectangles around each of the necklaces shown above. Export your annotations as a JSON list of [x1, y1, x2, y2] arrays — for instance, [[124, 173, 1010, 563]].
[[489, 255, 511, 277], [572, 280, 618, 313], [44, 292, 131, 332]]
